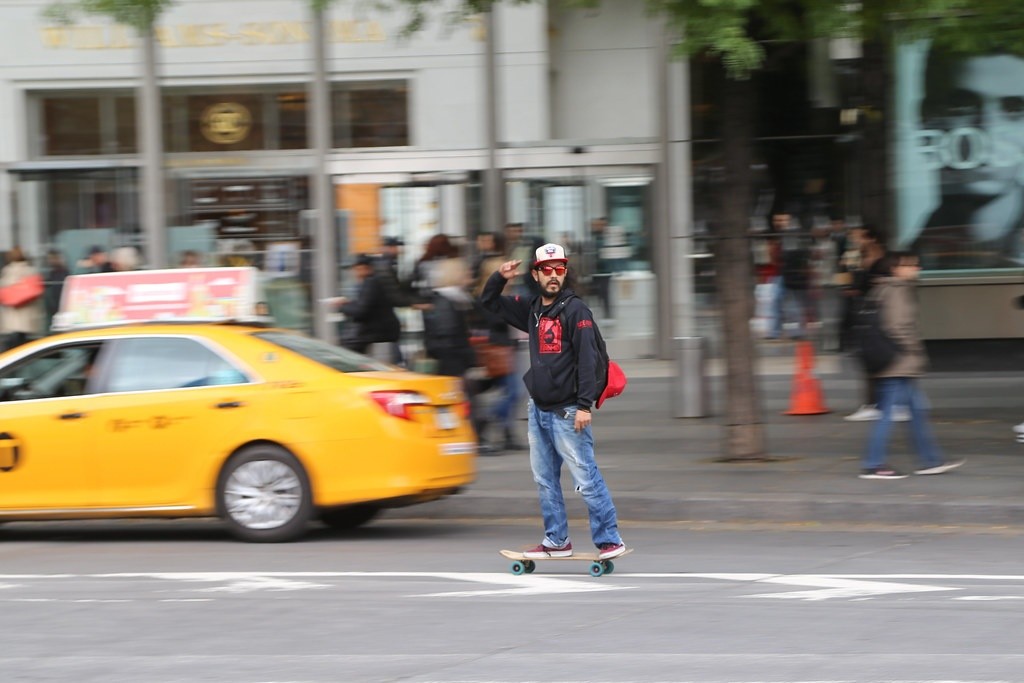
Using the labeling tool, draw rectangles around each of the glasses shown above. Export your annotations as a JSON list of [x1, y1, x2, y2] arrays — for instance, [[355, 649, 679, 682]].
[[536, 265, 566, 276]]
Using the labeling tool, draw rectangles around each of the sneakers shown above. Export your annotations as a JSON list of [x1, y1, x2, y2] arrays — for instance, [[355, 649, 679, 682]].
[[524, 542, 573, 558], [912, 456, 965, 474], [858, 467, 907, 479], [599, 543, 626, 559]]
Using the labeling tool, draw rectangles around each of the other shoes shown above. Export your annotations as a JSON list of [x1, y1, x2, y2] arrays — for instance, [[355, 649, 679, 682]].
[[890, 404, 912, 421], [503, 425, 527, 449], [473, 419, 499, 455], [844, 404, 882, 421]]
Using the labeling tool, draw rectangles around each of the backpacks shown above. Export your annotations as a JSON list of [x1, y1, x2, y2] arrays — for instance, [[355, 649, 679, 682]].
[[559, 299, 609, 401], [836, 292, 896, 375]]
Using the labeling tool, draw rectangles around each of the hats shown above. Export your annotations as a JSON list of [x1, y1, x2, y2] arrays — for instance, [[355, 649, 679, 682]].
[[341, 253, 374, 269], [533, 243, 567, 265], [380, 235, 404, 246], [595, 360, 626, 409]]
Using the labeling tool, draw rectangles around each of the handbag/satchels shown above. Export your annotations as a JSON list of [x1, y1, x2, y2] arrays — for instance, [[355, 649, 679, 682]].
[[0, 275, 45, 307]]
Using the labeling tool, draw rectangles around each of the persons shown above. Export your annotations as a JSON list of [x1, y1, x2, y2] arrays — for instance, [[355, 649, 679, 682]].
[[907, 34, 1024, 271], [179, 250, 202, 267], [479, 243, 625, 560], [843, 231, 912, 420], [0, 247, 43, 348], [332, 224, 542, 456], [44, 244, 141, 313], [859, 252, 966, 479], [765, 211, 873, 354]]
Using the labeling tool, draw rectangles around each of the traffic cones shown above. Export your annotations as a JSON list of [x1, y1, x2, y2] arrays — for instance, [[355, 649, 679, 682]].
[[779, 341, 835, 415]]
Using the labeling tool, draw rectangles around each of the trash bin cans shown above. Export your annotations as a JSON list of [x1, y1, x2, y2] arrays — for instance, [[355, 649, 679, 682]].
[[669, 336, 711, 418]]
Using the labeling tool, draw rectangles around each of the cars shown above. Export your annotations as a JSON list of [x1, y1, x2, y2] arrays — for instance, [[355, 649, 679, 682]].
[[0, 267, 478, 546]]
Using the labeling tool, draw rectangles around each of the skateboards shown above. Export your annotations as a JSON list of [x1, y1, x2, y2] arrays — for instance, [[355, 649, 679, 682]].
[[499, 549, 635, 577]]
